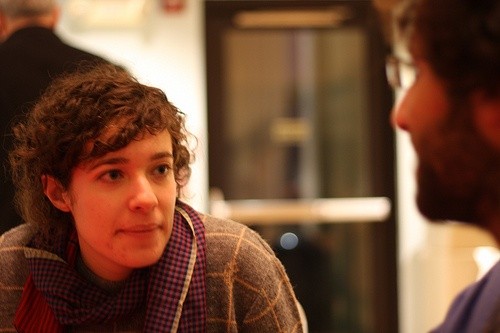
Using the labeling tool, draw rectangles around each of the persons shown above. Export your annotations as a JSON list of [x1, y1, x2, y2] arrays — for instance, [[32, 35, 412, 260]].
[[377, 0, 500, 333], [0, 0, 122, 239], [0, 63, 308, 333]]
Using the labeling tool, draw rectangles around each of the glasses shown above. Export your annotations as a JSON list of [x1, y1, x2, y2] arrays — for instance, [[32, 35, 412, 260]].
[[384, 52, 442, 94]]
